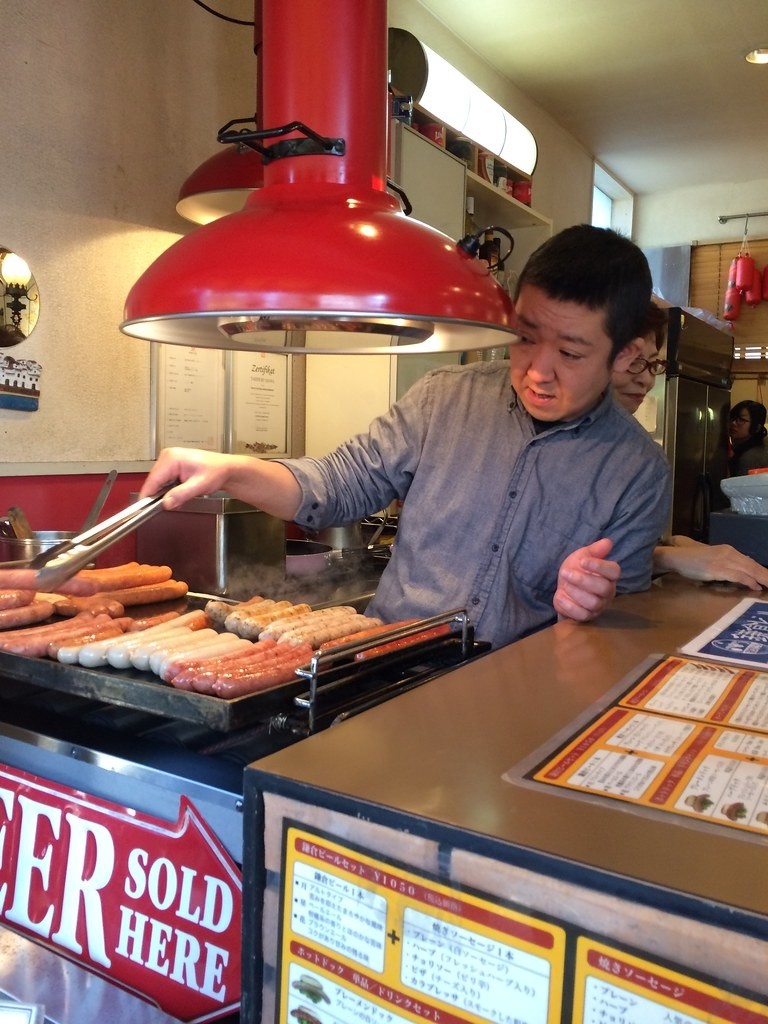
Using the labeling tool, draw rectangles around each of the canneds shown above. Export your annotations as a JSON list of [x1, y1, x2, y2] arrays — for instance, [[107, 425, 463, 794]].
[[413, 122, 530, 207]]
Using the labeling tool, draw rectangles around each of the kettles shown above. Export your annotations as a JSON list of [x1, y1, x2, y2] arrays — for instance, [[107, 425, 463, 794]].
[[298, 508, 388, 549]]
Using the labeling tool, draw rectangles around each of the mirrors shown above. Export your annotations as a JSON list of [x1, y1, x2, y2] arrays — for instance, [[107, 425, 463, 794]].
[[1, 246, 41, 349]]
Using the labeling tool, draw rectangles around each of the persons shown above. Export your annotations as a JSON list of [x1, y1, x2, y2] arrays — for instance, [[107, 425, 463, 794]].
[[137, 224, 674, 654], [612, 301, 768, 592], [730, 400, 768, 477]]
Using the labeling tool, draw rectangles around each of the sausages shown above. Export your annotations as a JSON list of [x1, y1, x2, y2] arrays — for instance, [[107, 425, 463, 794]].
[[0, 562, 450, 700]]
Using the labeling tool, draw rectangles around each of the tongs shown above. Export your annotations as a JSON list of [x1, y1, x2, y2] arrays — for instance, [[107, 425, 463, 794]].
[[27, 480, 181, 593]]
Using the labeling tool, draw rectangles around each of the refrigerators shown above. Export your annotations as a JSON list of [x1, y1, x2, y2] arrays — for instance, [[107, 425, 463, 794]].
[[633, 306, 736, 540]]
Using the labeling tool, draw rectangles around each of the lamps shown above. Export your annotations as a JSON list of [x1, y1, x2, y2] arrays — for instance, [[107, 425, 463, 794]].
[[1, 252, 38, 333], [739, 43, 768, 64], [388, 25, 539, 175]]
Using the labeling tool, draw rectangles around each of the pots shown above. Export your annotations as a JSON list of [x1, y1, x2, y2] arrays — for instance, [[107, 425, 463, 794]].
[[285, 539, 352, 575]]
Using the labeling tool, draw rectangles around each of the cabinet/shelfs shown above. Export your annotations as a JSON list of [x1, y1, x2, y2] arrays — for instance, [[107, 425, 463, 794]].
[[304, 116, 554, 518]]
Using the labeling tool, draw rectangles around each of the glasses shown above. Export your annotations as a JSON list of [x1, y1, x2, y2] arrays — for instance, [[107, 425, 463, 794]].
[[626, 358, 671, 375], [727, 416, 751, 426]]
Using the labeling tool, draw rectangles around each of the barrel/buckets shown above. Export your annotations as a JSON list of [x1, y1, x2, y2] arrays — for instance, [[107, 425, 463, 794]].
[[0, 530, 79, 561]]
